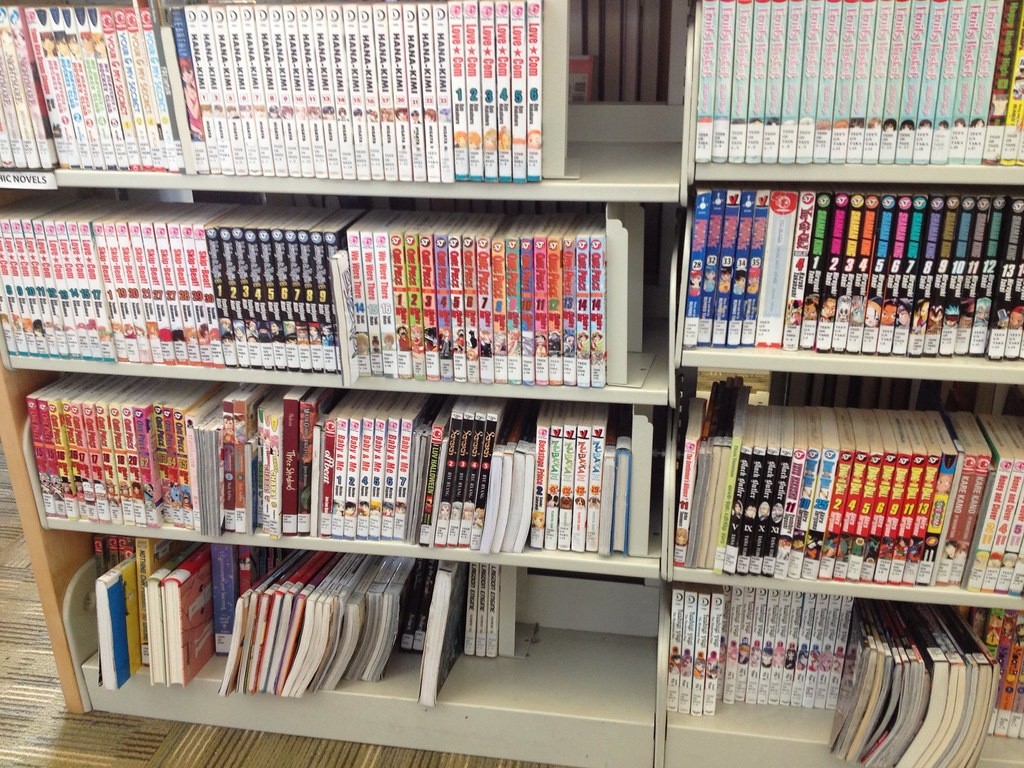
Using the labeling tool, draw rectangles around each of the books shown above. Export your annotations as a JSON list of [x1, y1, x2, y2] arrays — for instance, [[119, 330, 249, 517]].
[[84, 532, 1024, 768], [0, 183, 1024, 391], [1, 0, 1024, 189], [21, 379, 1024, 598]]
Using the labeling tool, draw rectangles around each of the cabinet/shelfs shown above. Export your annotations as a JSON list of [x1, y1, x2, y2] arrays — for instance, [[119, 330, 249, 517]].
[[0, 0, 1024, 768]]
